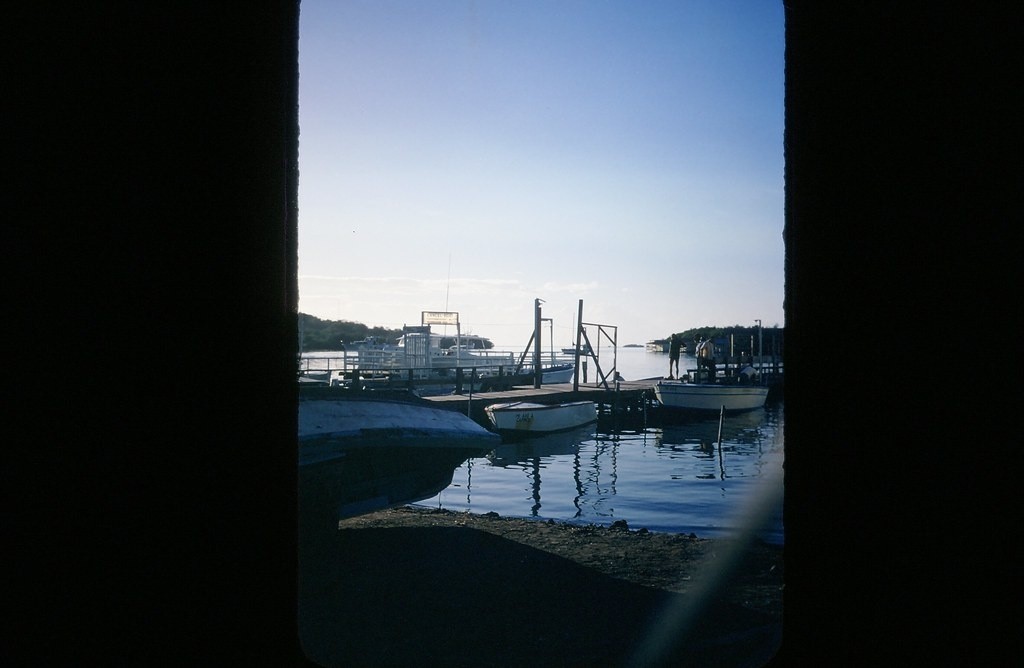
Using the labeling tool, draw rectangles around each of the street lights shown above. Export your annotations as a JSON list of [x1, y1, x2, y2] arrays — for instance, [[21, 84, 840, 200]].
[[755, 319, 762, 382]]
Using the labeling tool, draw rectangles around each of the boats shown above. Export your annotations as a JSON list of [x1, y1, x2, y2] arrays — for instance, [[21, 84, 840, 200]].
[[485, 420, 597, 466], [561, 344, 591, 355], [339, 333, 574, 397], [653, 382, 769, 412], [484, 401, 598, 435]]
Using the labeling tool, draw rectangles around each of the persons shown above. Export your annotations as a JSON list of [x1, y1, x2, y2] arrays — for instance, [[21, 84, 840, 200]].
[[730, 366, 758, 385], [696, 336, 715, 377], [668, 333, 687, 380]]
